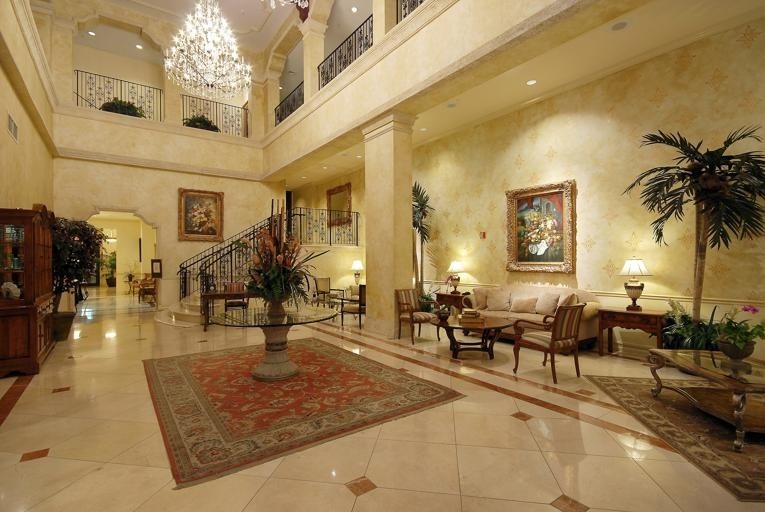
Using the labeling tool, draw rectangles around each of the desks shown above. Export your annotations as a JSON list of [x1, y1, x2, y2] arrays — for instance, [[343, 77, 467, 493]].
[[433, 290, 474, 315], [207, 306, 340, 383], [200, 291, 264, 332], [595, 305, 669, 358]]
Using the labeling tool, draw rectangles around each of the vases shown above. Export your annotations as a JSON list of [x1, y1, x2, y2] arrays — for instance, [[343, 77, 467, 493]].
[[258, 294, 292, 318], [714, 340, 759, 361]]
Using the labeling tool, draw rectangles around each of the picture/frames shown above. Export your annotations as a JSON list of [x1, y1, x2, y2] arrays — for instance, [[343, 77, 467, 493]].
[[504, 179, 578, 276], [151, 258, 163, 279], [326, 181, 352, 228], [177, 188, 225, 242]]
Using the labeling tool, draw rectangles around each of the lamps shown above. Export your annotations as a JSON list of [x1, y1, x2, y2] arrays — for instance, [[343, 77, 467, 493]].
[[162, 0, 254, 102], [616, 255, 653, 312], [447, 260, 466, 294], [351, 258, 364, 285]]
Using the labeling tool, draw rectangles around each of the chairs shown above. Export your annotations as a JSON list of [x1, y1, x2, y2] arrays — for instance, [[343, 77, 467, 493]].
[[221, 281, 249, 318], [341, 284, 366, 330], [395, 288, 442, 346], [313, 277, 345, 309], [512, 302, 588, 385], [128, 273, 158, 304]]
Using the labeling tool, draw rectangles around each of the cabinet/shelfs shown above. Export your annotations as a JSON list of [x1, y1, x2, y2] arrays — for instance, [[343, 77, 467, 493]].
[[0, 204, 56, 375]]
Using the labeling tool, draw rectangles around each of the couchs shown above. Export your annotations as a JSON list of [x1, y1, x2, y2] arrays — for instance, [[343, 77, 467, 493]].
[[461, 285, 602, 357]]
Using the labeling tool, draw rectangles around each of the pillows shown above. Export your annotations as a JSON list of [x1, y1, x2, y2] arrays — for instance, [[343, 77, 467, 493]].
[[484, 287, 512, 313], [509, 297, 539, 315], [556, 293, 579, 313], [472, 286, 491, 309], [534, 291, 561, 317]]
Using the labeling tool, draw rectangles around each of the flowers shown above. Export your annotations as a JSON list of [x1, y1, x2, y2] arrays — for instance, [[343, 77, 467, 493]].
[[230, 198, 331, 313], [711, 303, 765, 348]]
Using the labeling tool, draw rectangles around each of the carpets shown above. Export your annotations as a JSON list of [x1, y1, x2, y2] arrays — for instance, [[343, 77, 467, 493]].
[[141, 336, 469, 492], [581, 373, 765, 505]]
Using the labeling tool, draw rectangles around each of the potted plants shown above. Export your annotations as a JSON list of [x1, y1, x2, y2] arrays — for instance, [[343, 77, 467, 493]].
[[96, 250, 116, 287], [120, 263, 138, 281], [51, 216, 111, 343]]
